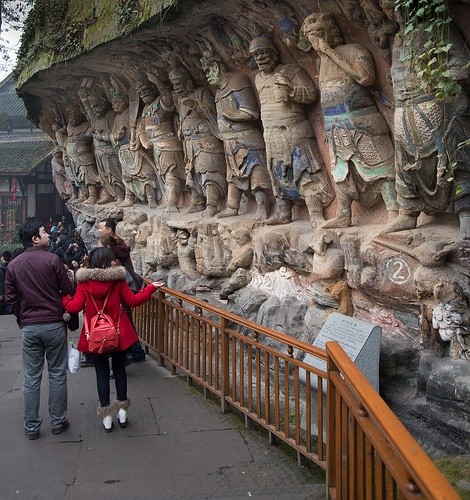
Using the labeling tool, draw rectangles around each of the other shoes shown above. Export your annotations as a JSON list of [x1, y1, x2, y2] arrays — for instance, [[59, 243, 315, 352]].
[[24, 428, 40, 440], [127, 354, 145, 364], [51, 417, 70, 435], [80, 358, 95, 368], [109, 358, 131, 380]]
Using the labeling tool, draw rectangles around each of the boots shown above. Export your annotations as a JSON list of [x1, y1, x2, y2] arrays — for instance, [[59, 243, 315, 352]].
[[96, 402, 115, 434], [112, 397, 131, 429]]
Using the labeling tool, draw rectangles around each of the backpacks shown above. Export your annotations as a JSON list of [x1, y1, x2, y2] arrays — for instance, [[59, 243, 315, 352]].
[[81, 279, 123, 354]]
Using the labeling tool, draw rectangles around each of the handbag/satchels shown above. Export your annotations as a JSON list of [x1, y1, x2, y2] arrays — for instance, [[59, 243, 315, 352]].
[[67, 329, 80, 374]]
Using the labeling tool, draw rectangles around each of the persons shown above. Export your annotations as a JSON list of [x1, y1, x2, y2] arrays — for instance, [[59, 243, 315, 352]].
[[53, 0, 470, 241], [59, 245, 166, 433], [0, 212, 145, 380], [5, 219, 77, 440]]
